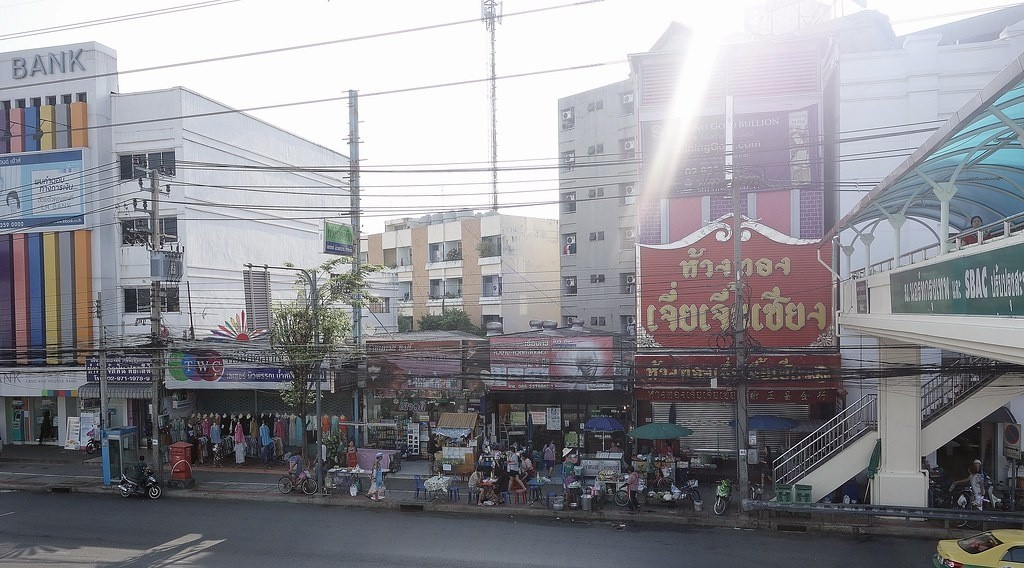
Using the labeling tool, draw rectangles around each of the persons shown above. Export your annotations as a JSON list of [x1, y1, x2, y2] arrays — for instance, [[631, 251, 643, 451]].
[[5, 191, 21, 219], [639, 439, 679, 462], [427, 433, 442, 475], [607, 441, 622, 453], [543, 435, 556, 478], [133, 456, 152, 486], [366, 354, 410, 388], [171, 412, 348, 464], [619, 466, 642, 510], [574, 345, 602, 390], [366, 452, 384, 500], [561, 445, 582, 508], [961, 216, 992, 246], [953, 459, 1000, 511], [465, 437, 535, 507]]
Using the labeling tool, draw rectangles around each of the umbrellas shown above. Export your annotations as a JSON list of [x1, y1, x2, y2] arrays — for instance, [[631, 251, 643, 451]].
[[863, 438, 882, 504], [584, 417, 625, 451], [627, 423, 693, 450], [729, 414, 800, 447]]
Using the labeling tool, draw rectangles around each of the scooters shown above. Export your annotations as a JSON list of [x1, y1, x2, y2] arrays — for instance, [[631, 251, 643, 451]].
[[985, 476, 1022, 512], [714, 479, 732, 516], [86, 424, 104, 454]]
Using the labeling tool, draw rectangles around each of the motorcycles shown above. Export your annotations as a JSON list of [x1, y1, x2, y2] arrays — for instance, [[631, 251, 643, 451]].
[[118, 456, 162, 500]]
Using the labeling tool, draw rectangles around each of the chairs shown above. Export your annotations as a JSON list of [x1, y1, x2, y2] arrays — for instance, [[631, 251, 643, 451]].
[[414, 474, 432, 501]]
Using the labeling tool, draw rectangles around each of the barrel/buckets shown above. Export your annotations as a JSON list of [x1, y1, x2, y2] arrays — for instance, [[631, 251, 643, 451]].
[[694, 500, 703, 511], [581, 494, 594, 510]]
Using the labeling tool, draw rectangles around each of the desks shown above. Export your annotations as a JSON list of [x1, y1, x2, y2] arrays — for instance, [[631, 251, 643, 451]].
[[525, 477, 547, 505], [689, 463, 717, 486], [328, 468, 392, 494], [423, 476, 452, 502], [353, 450, 401, 471]]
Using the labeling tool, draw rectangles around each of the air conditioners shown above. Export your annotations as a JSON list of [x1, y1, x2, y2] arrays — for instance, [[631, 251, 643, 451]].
[[626, 317, 635, 325], [565, 154, 575, 163], [566, 236, 575, 245], [567, 317, 577, 325], [623, 94, 634, 103], [625, 186, 637, 196], [566, 195, 575, 203], [563, 111, 571, 120], [626, 275, 635, 284], [625, 230, 635, 239], [565, 279, 575, 287], [625, 141, 634, 150]]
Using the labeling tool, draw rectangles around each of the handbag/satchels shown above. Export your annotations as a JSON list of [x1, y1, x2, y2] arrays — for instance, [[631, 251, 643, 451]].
[[368, 481, 378, 495]]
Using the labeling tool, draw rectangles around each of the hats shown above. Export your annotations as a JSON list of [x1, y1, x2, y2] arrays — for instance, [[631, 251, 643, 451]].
[[476, 467, 484, 471], [375, 452, 382, 459], [561, 448, 572, 457]]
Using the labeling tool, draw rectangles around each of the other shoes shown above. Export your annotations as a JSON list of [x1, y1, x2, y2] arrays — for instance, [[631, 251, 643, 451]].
[[637, 505, 641, 509], [626, 508, 631, 511], [478, 503, 483, 506], [546, 475, 551, 478]]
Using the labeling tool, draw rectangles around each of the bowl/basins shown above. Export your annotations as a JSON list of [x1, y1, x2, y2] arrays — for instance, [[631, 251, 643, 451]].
[[569, 503, 577, 509]]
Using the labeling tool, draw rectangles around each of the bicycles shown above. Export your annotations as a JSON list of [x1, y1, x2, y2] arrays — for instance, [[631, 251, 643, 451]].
[[646, 476, 702, 504], [748, 481, 765, 500], [927, 487, 982, 528], [278, 467, 318, 495]]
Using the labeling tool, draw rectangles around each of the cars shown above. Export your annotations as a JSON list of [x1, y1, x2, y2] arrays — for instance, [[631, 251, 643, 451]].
[[931, 529, 1024, 568]]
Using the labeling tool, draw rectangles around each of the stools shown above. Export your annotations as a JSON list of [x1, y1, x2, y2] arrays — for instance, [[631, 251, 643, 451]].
[[460, 470, 473, 483], [449, 487, 558, 505]]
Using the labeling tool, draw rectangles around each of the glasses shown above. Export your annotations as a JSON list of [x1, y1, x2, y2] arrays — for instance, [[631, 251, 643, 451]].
[[974, 463, 979, 465]]
